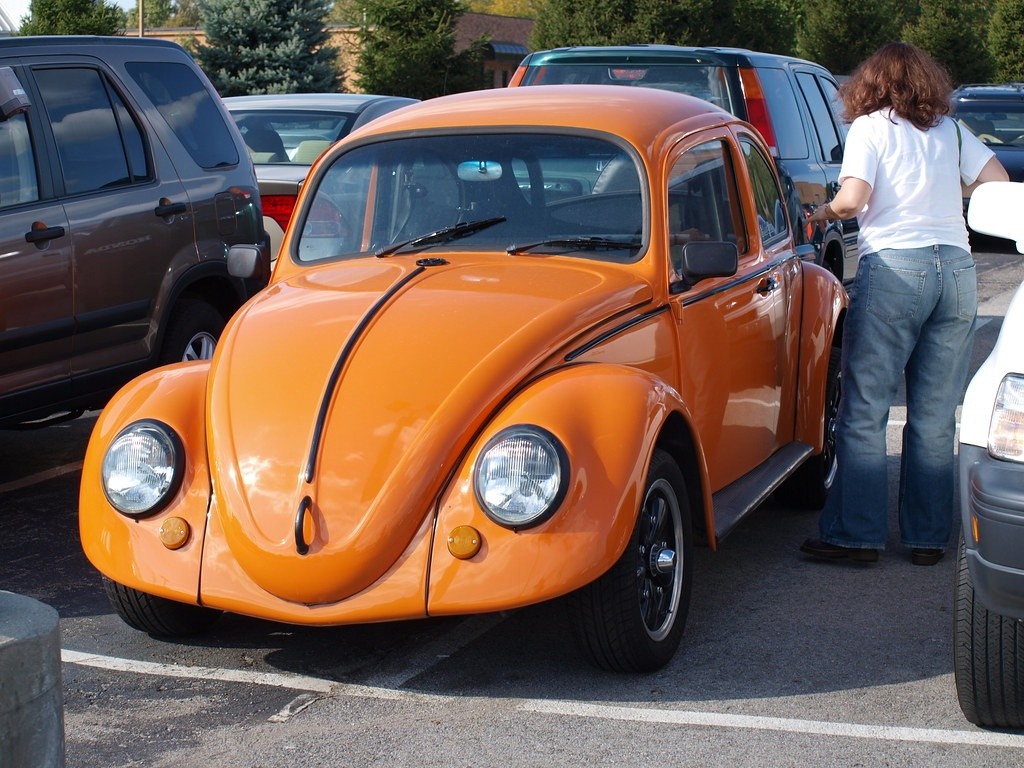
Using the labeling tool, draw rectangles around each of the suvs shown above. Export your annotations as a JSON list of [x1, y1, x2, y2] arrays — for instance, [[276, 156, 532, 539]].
[[0, 34, 273, 494], [955, 180, 1024, 731], [505, 45, 858, 291]]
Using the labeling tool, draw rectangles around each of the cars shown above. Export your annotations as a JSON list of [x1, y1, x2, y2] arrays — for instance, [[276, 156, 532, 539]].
[[275, 128, 334, 163], [219, 94, 422, 275], [79, 83, 849, 669], [946, 84, 1023, 230]]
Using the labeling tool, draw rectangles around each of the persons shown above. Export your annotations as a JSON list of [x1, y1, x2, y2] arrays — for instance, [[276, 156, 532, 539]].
[[670, 227, 705, 246], [798, 42, 1012, 568]]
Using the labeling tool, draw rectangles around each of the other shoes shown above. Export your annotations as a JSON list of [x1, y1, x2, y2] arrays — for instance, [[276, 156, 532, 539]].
[[911, 548, 940, 565], [799, 536, 878, 562]]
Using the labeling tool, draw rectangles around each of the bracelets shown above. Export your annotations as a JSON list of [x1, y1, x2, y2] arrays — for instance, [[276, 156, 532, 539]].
[[673, 233, 677, 245], [825, 205, 831, 218]]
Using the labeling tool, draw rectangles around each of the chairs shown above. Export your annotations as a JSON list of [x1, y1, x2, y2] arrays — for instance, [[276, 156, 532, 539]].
[[976, 120, 997, 134], [296, 140, 333, 164]]
